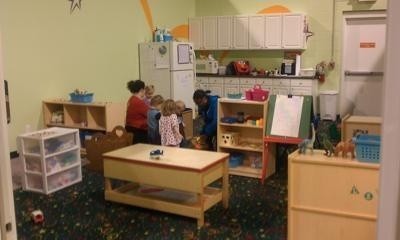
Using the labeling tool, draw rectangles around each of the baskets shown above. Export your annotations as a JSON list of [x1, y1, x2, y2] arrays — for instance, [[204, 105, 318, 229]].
[[352, 133, 379, 162], [85, 126, 135, 173], [246, 84, 269, 101]]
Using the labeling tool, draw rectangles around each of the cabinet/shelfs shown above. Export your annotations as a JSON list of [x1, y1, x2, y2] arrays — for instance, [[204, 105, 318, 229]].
[[218, 15, 249, 50], [249, 14, 282, 50], [287, 149, 379, 240], [282, 13, 313, 50], [341, 115, 382, 144], [19, 127, 83, 194], [224, 77, 240, 99], [217, 99, 276, 179], [208, 77, 224, 98], [291, 78, 317, 117], [43, 101, 125, 156], [255, 78, 272, 95], [272, 78, 290, 95], [240, 78, 255, 100], [197, 77, 208, 97], [189, 16, 217, 50]]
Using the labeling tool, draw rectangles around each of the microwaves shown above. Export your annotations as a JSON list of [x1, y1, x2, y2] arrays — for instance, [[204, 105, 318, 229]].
[[194, 59, 220, 77]]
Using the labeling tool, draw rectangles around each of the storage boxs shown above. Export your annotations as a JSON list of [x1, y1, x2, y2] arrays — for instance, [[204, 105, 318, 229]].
[[300, 69, 316, 76]]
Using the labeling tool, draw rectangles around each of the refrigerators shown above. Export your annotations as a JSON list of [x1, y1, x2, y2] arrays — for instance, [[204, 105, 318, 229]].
[[139, 41, 196, 120]]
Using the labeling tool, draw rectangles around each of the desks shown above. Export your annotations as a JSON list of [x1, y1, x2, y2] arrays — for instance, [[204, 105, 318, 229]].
[[102, 143, 230, 228]]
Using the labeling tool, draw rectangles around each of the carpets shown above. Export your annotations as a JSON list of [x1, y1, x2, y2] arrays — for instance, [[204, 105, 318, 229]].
[[13, 169, 286, 240]]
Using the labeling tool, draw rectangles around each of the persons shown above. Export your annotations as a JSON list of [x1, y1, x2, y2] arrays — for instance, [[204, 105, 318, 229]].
[[172, 101, 185, 146], [193, 89, 220, 150], [126, 80, 150, 142], [146, 95, 164, 144], [142, 85, 154, 104], [159, 99, 183, 146]]
